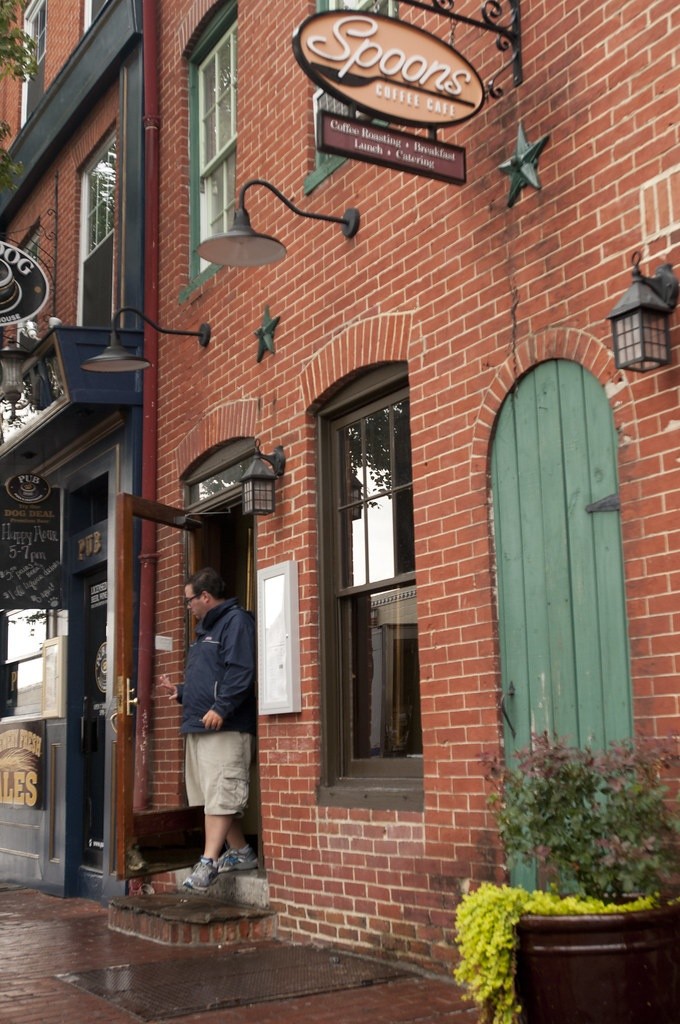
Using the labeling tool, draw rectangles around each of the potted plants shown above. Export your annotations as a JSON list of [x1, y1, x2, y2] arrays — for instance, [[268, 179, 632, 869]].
[[451, 728, 680, 1024]]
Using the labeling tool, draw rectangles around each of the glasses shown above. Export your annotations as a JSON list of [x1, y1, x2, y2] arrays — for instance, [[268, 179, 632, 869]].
[[185, 595, 201, 606]]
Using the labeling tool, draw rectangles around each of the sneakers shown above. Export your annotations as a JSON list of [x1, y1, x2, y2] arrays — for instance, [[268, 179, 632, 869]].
[[217, 845, 258, 873], [183, 856, 220, 892]]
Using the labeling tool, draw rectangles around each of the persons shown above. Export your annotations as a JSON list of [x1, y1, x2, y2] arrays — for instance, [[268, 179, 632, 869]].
[[156, 571, 256, 890]]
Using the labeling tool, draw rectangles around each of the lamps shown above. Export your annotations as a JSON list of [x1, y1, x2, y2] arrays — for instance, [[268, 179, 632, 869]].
[[240, 436, 287, 516], [605, 251, 679, 375], [79, 306, 211, 373], [195, 179, 361, 266], [0, 334, 31, 424]]
